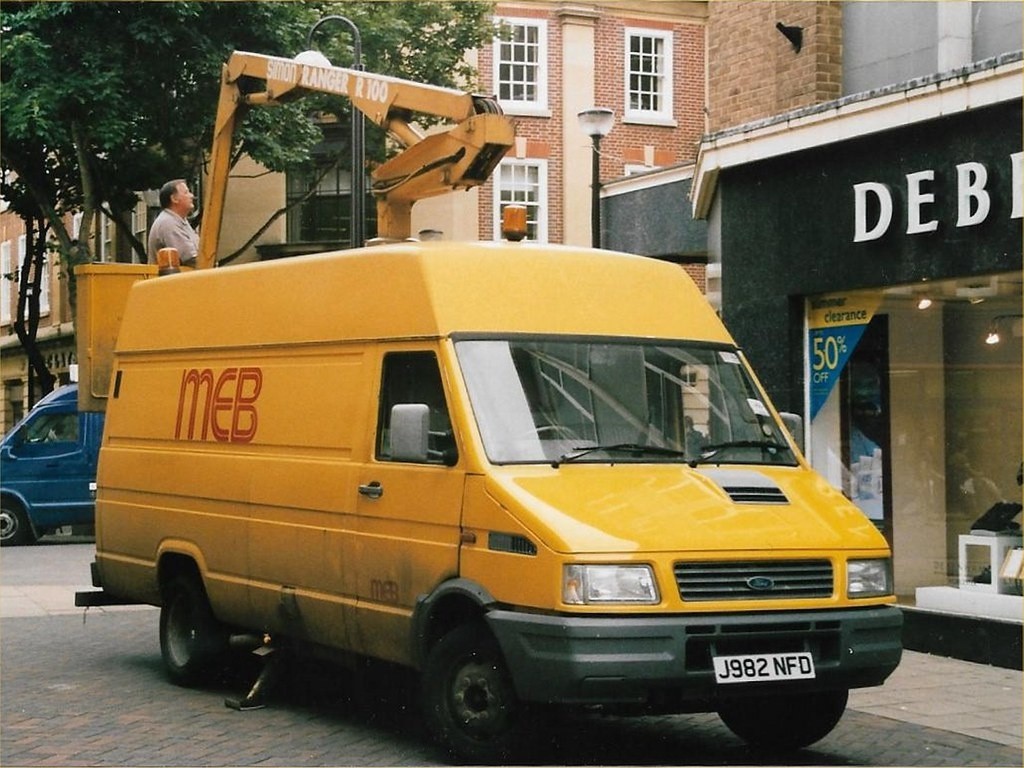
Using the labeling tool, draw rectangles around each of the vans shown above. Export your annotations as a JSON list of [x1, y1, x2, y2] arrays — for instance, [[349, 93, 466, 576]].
[[74, 240, 907, 766], [0, 382, 106, 548]]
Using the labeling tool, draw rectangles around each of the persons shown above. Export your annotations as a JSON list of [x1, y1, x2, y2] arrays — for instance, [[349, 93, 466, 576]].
[[148, 180, 200, 267]]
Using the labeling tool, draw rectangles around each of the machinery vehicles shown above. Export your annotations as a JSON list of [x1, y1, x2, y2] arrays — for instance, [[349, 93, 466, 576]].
[[74, 50, 517, 415]]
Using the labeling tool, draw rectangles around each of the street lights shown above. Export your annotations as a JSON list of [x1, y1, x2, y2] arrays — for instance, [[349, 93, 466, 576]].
[[291, 16, 365, 251], [577, 109, 616, 248]]
